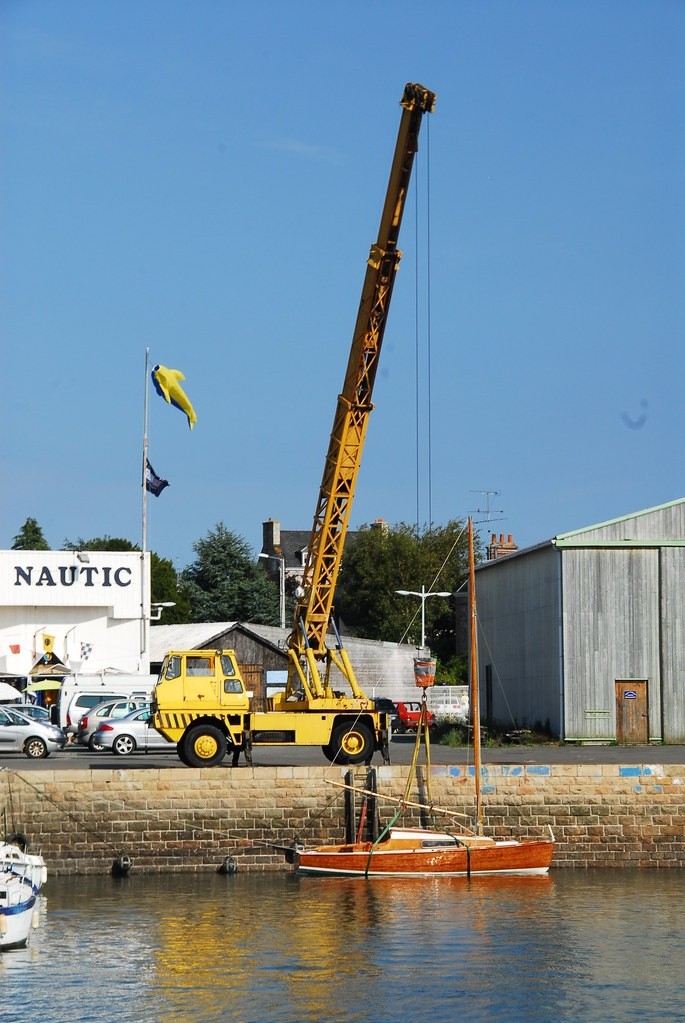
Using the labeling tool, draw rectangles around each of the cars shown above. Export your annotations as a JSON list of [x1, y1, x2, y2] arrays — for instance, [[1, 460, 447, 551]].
[[391, 702, 436, 732], [437, 697, 466, 721], [0, 705, 65, 759], [96, 707, 180, 755], [5, 704, 51, 724], [77, 699, 154, 751]]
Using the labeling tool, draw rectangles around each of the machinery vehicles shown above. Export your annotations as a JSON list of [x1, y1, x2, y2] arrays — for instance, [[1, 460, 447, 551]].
[[145, 84, 438, 768]]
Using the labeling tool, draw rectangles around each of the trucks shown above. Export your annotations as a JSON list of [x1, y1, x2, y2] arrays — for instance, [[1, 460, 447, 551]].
[[52, 675, 159, 736]]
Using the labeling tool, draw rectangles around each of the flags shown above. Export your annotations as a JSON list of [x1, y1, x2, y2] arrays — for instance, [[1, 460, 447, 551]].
[[143, 452, 170, 497]]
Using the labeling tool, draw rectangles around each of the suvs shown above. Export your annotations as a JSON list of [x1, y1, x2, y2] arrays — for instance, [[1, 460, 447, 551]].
[[66, 691, 156, 735]]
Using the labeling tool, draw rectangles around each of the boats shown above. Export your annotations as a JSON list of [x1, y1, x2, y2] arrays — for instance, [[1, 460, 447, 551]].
[[277, 516, 553, 877], [1, 832, 49, 950]]
